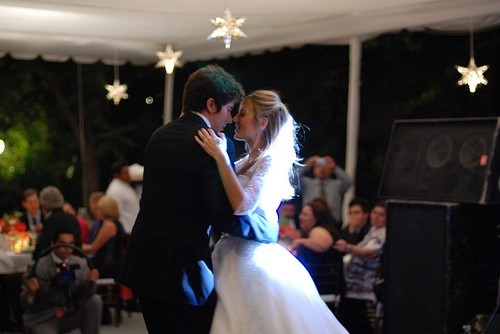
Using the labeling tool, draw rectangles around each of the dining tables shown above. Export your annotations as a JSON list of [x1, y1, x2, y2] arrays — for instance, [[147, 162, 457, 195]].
[[0, 231, 41, 333]]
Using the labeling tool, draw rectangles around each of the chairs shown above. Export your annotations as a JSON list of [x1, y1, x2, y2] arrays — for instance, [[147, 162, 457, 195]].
[[95, 233, 131, 327], [297, 245, 347, 306]]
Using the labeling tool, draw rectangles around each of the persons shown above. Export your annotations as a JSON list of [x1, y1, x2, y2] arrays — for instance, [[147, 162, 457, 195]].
[[25, 185, 82, 281], [82, 197, 125, 326], [26, 224, 102, 333], [114, 65, 280, 334], [278, 196, 387, 306], [299, 156, 352, 229], [22, 163, 143, 252], [195, 90, 352, 334]]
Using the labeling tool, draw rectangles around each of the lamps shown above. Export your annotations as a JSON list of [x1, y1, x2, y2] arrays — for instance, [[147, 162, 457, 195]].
[[154, 16, 185, 74], [207, 0, 247, 48], [105, 39, 128, 105], [456, 16, 489, 93]]
